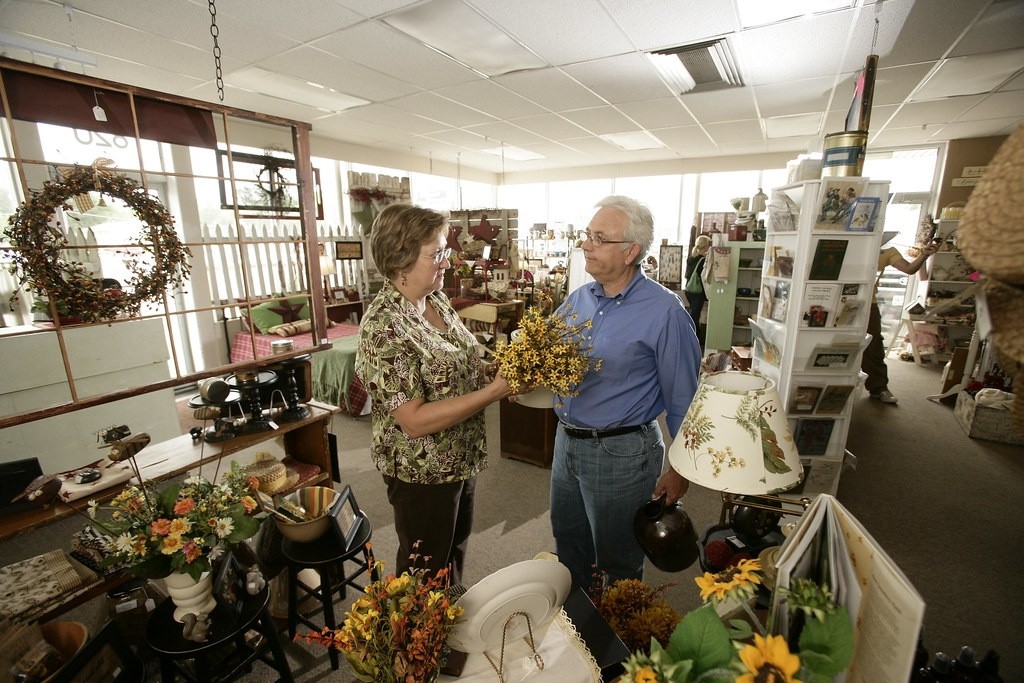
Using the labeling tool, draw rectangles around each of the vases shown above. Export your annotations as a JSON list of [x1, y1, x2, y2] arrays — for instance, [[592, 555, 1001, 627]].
[[163, 557, 218, 623]]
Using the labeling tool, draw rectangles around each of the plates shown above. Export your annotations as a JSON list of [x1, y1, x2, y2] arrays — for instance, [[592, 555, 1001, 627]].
[[445, 559, 572, 654]]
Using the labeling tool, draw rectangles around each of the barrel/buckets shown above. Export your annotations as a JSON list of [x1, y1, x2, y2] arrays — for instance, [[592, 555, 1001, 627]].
[[820, 130, 869, 178]]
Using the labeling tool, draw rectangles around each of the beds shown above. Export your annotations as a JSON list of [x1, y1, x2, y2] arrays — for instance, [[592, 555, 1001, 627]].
[[228, 291, 373, 418]]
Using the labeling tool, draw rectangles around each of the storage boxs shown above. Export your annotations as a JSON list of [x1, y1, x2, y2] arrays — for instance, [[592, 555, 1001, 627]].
[[952, 391, 1024, 446]]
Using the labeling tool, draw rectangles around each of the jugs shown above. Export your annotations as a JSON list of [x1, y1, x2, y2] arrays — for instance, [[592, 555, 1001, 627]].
[[632, 492, 700, 573]]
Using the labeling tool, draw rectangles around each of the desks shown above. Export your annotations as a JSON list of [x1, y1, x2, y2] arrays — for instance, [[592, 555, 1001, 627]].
[[452, 259, 508, 304], [449, 296, 525, 351], [0, 317, 182, 476], [426, 579, 633, 683], [0, 399, 381, 683]]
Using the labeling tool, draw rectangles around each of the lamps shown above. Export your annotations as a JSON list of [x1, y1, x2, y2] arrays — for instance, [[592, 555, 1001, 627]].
[[319, 255, 337, 304], [667, 372, 806, 579]]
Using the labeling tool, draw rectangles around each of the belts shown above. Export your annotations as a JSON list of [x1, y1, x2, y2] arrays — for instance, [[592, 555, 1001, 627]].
[[563, 422, 650, 439]]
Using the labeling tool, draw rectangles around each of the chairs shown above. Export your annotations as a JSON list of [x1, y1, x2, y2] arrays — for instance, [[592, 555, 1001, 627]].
[[508, 268, 534, 310]]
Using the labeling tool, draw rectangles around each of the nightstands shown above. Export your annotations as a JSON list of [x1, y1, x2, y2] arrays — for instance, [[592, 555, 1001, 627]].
[[324, 301, 363, 327]]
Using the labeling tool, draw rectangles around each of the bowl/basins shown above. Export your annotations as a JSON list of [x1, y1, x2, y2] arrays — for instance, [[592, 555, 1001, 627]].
[[270, 486, 338, 543], [542, 235, 548, 240]]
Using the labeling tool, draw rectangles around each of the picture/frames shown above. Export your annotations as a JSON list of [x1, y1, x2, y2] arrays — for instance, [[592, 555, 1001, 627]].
[[331, 289, 347, 299], [335, 241, 363, 260]]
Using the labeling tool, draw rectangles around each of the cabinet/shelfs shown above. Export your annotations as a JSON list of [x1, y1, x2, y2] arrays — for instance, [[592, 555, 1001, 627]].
[[749, 175, 892, 528], [916, 218, 976, 364], [515, 237, 577, 298], [703, 241, 766, 358]]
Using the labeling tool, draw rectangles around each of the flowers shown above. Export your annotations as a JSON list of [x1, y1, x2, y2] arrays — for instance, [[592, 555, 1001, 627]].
[[488, 301, 605, 412], [619, 559, 857, 683], [291, 539, 468, 683], [71, 464, 277, 584]]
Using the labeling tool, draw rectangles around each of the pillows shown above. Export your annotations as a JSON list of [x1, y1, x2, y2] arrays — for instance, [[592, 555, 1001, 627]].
[[268, 319, 310, 335], [234, 296, 273, 332], [240, 294, 312, 336], [275, 318, 336, 337]]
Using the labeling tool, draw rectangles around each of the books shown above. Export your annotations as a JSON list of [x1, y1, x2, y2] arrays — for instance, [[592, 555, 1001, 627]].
[[767, 493, 925, 683]]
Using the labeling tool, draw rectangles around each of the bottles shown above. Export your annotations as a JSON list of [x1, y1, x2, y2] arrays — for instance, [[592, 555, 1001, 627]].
[[909, 624, 1006, 683], [272, 339, 294, 354]]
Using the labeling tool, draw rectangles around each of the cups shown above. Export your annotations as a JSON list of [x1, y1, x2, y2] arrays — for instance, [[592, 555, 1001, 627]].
[[533, 230, 566, 257]]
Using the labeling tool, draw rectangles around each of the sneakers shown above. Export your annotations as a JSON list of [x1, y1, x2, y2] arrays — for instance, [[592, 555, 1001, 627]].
[[870, 390, 898, 402]]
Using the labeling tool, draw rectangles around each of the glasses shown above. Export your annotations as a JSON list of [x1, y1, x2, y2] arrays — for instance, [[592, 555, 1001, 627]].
[[580, 232, 633, 247], [420, 248, 452, 263]]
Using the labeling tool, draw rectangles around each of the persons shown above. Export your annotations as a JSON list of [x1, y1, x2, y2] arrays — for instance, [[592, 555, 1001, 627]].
[[860, 239, 945, 402], [545, 194, 703, 602], [685, 235, 710, 344], [354, 205, 537, 593]]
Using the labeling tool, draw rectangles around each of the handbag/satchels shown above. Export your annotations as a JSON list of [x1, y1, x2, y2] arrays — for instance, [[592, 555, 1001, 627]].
[[686, 257, 706, 294]]
